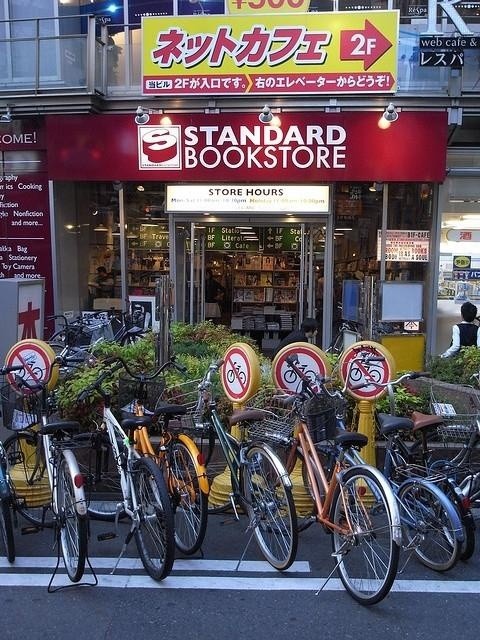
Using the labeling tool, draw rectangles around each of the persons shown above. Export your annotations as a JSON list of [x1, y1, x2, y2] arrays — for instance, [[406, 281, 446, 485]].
[[90, 266, 114, 298], [274, 319, 318, 357], [438, 302, 480, 358], [206, 269, 225, 303], [316, 268, 355, 330]]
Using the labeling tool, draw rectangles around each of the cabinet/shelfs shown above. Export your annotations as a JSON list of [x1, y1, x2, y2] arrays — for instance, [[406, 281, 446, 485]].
[[87, 248, 415, 359]]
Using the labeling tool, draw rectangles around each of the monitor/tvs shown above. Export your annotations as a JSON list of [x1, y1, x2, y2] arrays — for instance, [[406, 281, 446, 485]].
[[375, 280, 425, 323], [341, 280, 363, 322]]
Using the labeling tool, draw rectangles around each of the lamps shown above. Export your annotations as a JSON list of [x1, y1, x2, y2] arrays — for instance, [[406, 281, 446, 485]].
[[141, 216, 159, 227], [134, 102, 399, 126], [93, 224, 109, 232]]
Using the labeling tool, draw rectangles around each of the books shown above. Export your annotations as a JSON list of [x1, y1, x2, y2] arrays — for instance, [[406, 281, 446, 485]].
[[102, 257, 170, 298], [205, 255, 232, 298], [233, 254, 300, 329], [335, 257, 409, 281]]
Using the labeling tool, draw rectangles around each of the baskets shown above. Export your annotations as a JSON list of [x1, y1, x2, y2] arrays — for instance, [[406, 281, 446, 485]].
[[430, 383, 479, 440], [65, 324, 93, 347], [244, 387, 349, 449], [118, 371, 208, 430], [0, 379, 52, 431], [123, 311, 146, 332]]
